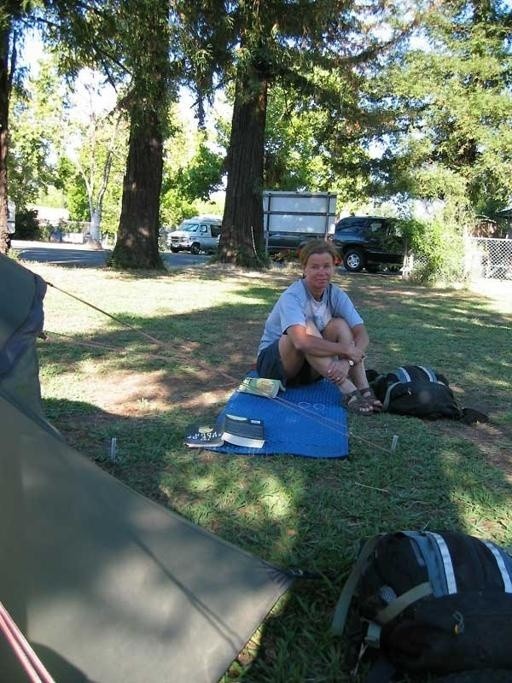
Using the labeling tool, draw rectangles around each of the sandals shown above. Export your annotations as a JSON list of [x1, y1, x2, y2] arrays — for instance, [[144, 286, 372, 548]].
[[341, 386, 382, 418]]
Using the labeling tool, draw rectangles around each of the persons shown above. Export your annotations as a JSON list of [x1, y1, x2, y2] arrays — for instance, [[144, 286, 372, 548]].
[[256, 237, 382, 414]]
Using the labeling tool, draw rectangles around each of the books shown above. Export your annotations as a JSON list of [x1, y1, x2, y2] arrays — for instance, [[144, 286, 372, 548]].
[[184, 411, 265, 448], [235, 375, 287, 398]]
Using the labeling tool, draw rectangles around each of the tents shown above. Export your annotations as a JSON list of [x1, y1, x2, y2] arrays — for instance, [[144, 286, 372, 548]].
[[0, 252, 302, 683]]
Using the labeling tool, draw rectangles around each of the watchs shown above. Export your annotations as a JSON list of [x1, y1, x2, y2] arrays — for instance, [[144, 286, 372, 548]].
[[346, 357, 355, 367]]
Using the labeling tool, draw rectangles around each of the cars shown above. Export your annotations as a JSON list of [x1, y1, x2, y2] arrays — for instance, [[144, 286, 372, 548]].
[[262, 230, 335, 262]]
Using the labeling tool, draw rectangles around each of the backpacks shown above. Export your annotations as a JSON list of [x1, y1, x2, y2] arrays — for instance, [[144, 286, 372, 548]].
[[340, 530, 512, 683], [366, 357, 468, 424]]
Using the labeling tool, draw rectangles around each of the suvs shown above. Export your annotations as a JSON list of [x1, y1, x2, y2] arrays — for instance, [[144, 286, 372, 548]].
[[330, 213, 427, 273], [165, 218, 223, 255]]
